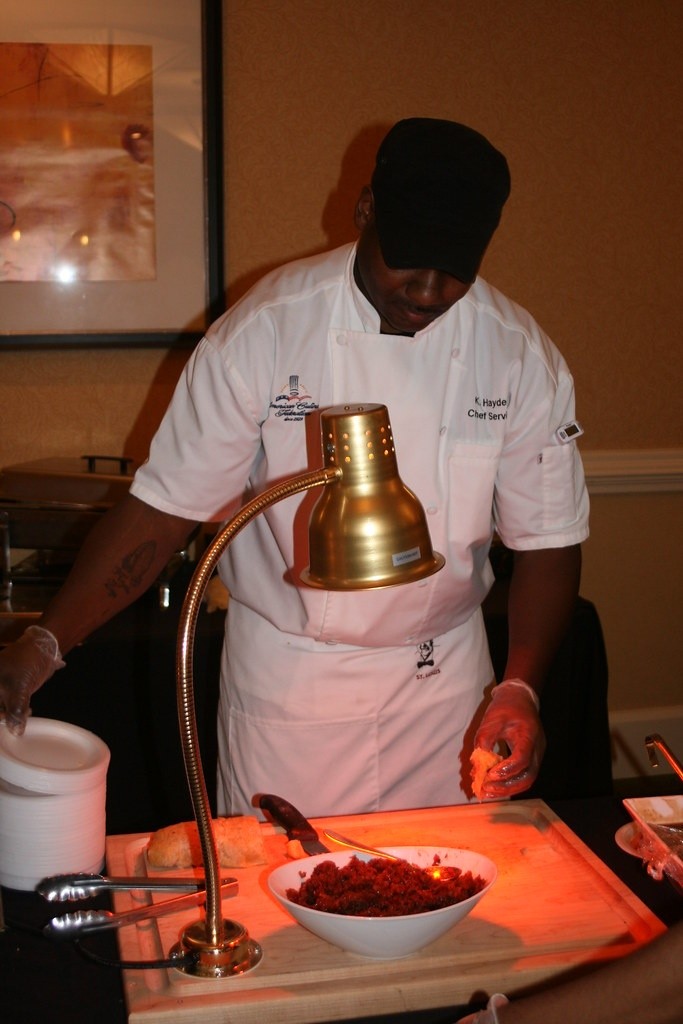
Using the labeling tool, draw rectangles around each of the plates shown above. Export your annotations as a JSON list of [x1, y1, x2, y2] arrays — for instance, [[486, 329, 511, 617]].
[[0, 717, 111, 893], [614, 821, 644, 858]]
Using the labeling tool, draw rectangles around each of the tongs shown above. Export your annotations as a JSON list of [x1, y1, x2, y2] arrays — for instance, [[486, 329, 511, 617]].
[[36, 872, 239, 940]]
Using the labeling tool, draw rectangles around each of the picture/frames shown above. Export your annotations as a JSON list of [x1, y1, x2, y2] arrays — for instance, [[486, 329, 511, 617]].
[[1, 0, 227, 353]]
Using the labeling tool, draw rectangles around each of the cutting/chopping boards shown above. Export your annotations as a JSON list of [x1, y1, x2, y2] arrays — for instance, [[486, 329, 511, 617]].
[[105, 798, 668, 1024]]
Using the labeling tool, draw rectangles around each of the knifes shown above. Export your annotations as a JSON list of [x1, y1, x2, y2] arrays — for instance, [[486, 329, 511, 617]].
[[258, 795, 331, 857]]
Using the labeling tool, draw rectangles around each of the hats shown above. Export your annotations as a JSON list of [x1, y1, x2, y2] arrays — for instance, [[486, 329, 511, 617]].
[[371, 115, 513, 286]]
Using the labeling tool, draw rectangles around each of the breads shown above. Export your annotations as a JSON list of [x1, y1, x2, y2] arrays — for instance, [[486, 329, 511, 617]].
[[469, 748, 502, 801], [147, 814, 268, 869], [286, 839, 304, 859]]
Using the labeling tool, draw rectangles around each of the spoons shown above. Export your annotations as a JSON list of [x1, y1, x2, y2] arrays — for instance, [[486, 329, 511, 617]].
[[324, 829, 463, 881]]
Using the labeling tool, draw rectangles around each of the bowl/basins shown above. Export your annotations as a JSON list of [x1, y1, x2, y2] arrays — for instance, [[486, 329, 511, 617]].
[[622, 795, 683, 885], [267, 844, 498, 961]]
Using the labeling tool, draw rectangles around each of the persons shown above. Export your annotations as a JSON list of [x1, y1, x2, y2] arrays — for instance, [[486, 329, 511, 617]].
[[0, 117, 591, 822]]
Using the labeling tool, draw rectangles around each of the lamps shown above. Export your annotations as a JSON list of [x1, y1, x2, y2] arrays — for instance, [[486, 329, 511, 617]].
[[166, 406, 446, 978]]
